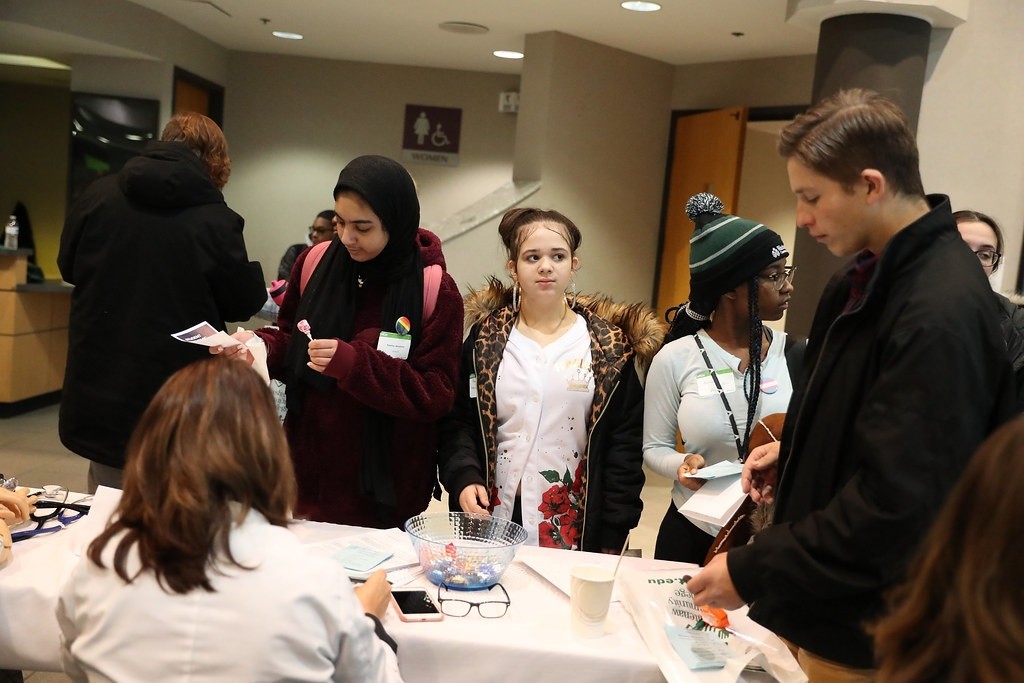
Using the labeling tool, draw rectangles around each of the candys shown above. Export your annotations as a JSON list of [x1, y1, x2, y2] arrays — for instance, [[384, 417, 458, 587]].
[[422, 543, 503, 586], [297, 319, 312, 341]]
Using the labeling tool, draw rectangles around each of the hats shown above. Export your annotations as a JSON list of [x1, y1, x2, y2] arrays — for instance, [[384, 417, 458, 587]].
[[685, 193, 789, 299]]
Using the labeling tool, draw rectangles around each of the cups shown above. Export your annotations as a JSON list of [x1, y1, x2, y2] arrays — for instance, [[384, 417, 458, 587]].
[[571, 568, 616, 627]]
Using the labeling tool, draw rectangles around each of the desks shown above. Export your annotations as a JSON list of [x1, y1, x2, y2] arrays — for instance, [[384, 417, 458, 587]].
[[0, 474, 806, 683]]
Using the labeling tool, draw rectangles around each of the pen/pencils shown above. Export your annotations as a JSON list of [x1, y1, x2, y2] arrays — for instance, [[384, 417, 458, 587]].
[[349, 578, 393, 585], [27, 492, 42, 497]]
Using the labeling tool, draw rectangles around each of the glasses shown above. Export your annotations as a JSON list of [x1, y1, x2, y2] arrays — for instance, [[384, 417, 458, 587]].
[[974, 249, 1001, 267], [309, 226, 332, 233], [438, 583, 510, 618], [29, 500, 90, 522], [759, 266, 795, 290]]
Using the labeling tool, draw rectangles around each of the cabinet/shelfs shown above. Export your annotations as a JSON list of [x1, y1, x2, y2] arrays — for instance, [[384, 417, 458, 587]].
[[0, 290, 70, 408]]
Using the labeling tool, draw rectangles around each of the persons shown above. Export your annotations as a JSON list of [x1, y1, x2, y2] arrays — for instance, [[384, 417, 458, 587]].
[[643, 192, 808, 567], [57, 111, 267, 495], [436, 208, 666, 557], [277, 210, 336, 281], [0, 358, 402, 683], [208, 155, 464, 532], [0, 486, 37, 526], [689, 88, 1024, 683]]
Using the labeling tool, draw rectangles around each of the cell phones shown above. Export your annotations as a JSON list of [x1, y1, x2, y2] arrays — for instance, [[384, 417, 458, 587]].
[[390, 588, 444, 622]]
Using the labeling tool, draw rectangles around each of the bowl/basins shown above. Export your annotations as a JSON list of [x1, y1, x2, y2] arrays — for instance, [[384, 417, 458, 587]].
[[405, 513, 527, 591]]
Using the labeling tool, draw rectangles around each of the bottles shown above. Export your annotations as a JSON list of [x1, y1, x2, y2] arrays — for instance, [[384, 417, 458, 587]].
[[4, 216, 19, 250], [269, 280, 289, 306]]
